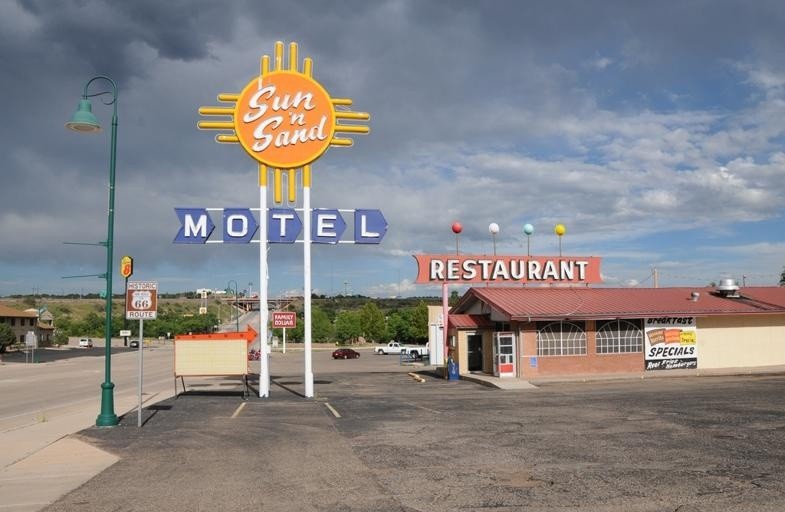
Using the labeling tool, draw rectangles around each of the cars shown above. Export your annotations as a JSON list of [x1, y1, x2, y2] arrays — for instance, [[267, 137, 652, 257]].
[[130, 341, 139, 348], [332, 348, 360, 359]]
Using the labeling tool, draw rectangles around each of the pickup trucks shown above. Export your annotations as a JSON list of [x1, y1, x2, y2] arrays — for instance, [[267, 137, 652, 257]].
[[407, 342, 429, 359], [374, 342, 407, 355]]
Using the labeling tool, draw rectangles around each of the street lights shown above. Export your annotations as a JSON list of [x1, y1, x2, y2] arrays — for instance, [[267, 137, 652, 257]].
[[64, 75, 118, 427], [224, 280, 239, 332]]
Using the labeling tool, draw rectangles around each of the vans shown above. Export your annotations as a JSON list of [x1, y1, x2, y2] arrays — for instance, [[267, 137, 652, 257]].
[[80, 338, 93, 347]]
